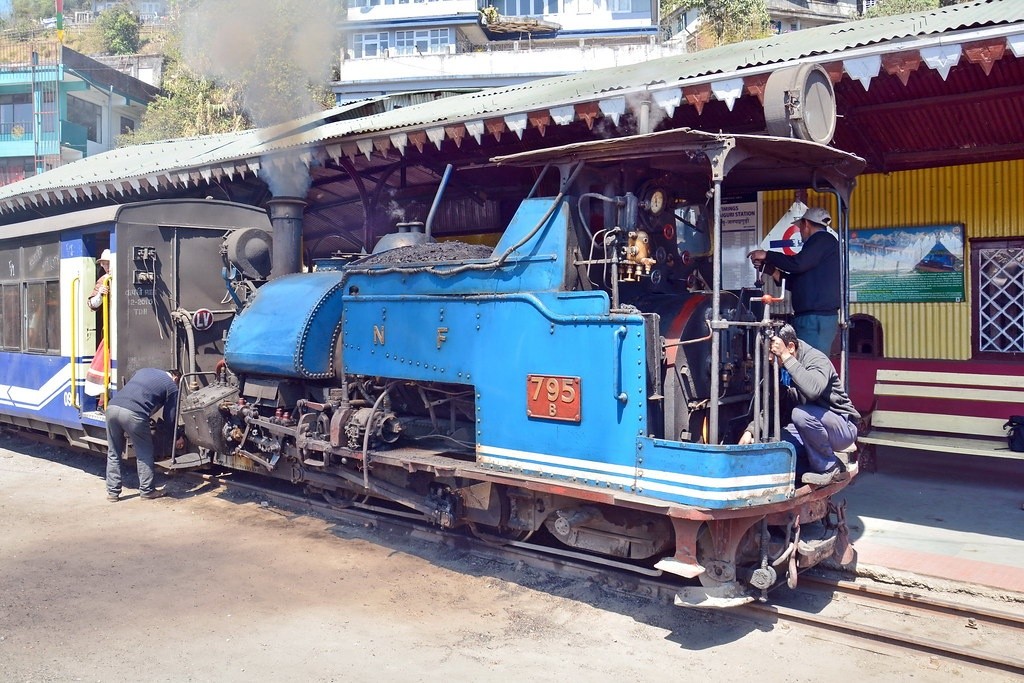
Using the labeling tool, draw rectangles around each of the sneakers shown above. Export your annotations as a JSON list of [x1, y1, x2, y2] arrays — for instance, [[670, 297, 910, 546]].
[[139, 488, 168, 500], [802, 465, 840, 486], [107, 496, 119, 502]]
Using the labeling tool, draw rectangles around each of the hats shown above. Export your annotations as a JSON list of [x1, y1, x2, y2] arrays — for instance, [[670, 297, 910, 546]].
[[96, 249, 111, 263], [791, 208, 831, 228]]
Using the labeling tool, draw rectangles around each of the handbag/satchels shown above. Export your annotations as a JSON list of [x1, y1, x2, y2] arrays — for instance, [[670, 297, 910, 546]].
[[1003, 416, 1024, 452]]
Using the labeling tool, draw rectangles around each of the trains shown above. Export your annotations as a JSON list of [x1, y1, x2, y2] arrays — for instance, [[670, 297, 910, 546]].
[[0, 127, 867, 614]]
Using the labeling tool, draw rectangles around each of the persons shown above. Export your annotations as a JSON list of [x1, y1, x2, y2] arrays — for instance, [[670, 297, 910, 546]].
[[105, 368, 182, 502], [738, 320, 862, 485], [747, 209, 840, 360], [85, 248, 110, 412]]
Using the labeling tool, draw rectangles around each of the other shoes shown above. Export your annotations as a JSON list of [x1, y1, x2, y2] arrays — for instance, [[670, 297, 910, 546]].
[[97, 401, 104, 411]]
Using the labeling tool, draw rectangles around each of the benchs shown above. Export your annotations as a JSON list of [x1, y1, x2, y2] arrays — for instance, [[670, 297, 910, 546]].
[[849, 370, 1024, 485]]
